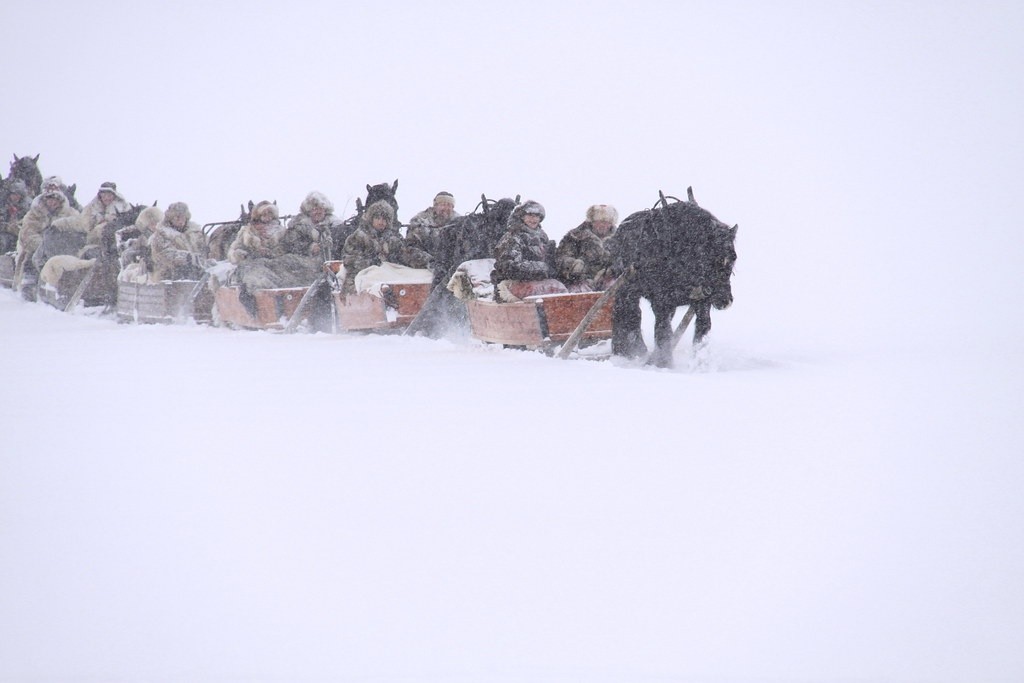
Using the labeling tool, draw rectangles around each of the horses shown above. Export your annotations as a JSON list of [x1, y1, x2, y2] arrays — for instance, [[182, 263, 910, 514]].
[[610, 200, 738, 369], [101, 200, 158, 315], [421, 198, 519, 339], [331, 178, 401, 260], [208, 204, 251, 262], [0, 153, 43, 235]]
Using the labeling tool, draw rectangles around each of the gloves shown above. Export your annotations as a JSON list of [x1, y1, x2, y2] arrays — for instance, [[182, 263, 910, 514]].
[[538, 262, 559, 279], [594, 269, 611, 290], [571, 259, 587, 284]]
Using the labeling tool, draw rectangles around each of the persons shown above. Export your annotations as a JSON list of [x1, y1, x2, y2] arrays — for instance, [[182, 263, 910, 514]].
[[490, 200, 555, 303], [1, 176, 464, 322], [553, 204, 619, 293]]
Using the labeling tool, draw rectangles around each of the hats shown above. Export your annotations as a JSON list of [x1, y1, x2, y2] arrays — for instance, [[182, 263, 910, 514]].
[[135, 207, 164, 232], [299, 191, 333, 216], [366, 200, 394, 224], [42, 182, 65, 204], [512, 201, 545, 223], [433, 191, 455, 208], [164, 202, 191, 223], [98, 182, 117, 196], [8, 178, 29, 197], [587, 204, 618, 224], [251, 200, 279, 221], [45, 176, 63, 188]]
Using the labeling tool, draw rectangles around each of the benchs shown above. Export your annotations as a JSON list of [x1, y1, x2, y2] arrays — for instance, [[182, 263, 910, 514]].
[[449, 258, 614, 348], [38, 227, 104, 309], [206, 222, 317, 329], [321, 260, 433, 332], [113, 224, 211, 324]]
